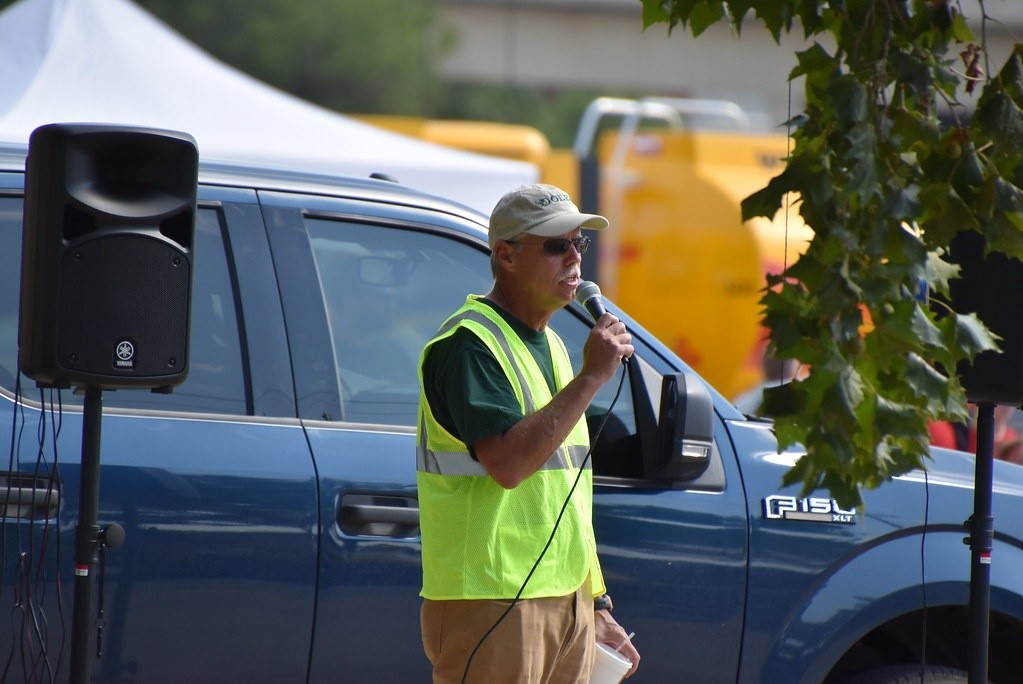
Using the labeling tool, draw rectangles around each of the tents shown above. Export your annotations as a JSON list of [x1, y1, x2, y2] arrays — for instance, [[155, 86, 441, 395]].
[[1, 1, 540, 217]]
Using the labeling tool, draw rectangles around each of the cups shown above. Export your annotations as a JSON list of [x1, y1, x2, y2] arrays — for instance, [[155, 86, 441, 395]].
[[588, 641, 633, 684]]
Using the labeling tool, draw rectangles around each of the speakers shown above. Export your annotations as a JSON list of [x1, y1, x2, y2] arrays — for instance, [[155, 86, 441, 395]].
[[16, 122, 200, 395], [926, 204, 1023, 404]]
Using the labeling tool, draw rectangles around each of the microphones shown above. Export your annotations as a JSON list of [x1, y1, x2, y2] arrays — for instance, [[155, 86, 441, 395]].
[[575, 281, 630, 365]]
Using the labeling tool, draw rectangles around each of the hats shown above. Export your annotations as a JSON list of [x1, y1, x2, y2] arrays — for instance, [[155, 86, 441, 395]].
[[487, 184, 609, 249]]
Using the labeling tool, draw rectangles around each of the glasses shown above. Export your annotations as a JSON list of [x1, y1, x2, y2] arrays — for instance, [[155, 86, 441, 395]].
[[505, 236, 591, 255]]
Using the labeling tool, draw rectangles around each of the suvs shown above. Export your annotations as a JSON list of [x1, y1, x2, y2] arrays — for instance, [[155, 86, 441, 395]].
[[0, 145, 1023, 684]]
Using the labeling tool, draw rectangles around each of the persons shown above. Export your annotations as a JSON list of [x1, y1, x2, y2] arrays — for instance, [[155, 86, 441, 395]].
[[415, 183, 641, 684]]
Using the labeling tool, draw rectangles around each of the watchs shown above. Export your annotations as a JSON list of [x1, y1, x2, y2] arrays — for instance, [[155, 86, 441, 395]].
[[594, 595, 613, 612]]
